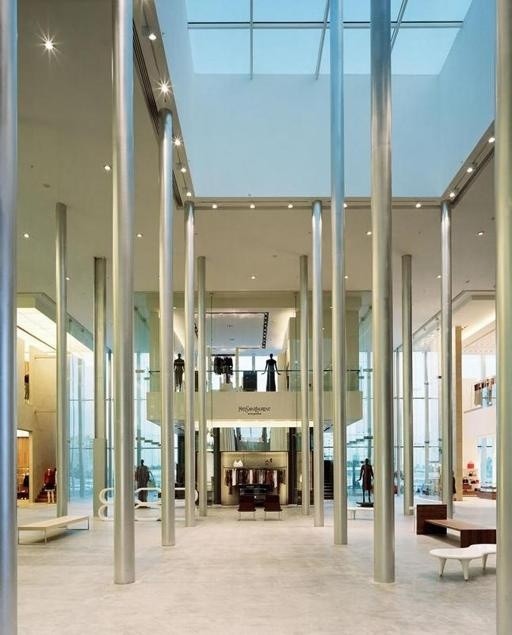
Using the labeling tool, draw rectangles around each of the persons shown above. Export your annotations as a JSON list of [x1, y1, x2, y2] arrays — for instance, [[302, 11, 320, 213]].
[[174, 353, 185, 392], [261, 353, 280, 392], [134, 458, 150, 502], [43, 468, 55, 504], [357, 458, 374, 503]]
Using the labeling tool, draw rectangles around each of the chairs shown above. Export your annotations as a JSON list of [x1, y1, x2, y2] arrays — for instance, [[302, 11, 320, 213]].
[[237, 494, 257, 522], [264, 495, 282, 521]]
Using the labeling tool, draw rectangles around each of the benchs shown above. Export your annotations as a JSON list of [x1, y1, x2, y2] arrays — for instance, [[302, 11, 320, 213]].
[[430, 544, 496, 580], [413, 503, 496, 548], [18, 513, 90, 545], [344, 505, 374, 521]]
